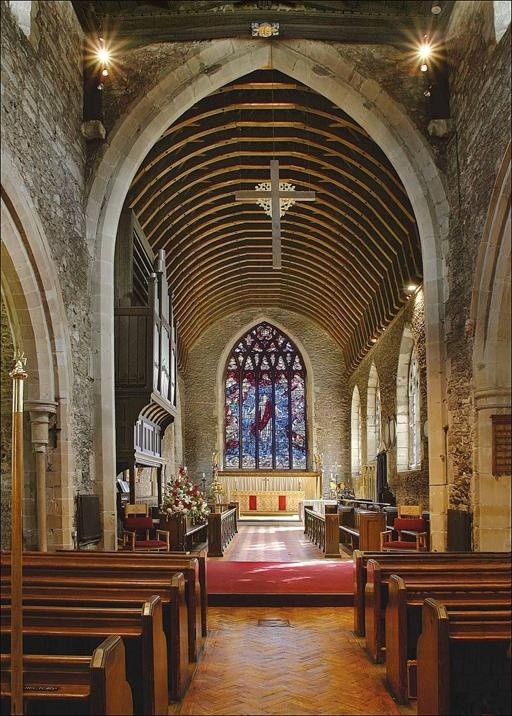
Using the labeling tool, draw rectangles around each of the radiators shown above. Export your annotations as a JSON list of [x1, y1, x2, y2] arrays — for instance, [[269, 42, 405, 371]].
[[74, 488, 102, 551]]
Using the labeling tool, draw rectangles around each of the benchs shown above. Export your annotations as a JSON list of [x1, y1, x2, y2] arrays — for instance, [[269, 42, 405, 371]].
[[339, 522, 360, 552], [185, 520, 209, 550]]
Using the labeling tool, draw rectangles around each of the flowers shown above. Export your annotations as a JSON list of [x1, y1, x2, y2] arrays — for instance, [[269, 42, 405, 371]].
[[162, 466, 211, 526]]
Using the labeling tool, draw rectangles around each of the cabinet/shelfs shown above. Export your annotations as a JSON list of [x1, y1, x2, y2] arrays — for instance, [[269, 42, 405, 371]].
[[124, 227, 157, 308]]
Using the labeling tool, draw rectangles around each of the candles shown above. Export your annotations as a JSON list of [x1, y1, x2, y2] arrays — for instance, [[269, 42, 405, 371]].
[[330, 473, 333, 479], [203, 473, 206, 477]]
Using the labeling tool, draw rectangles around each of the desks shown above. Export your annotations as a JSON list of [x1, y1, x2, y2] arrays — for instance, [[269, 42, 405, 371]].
[[1, 546, 206, 716], [350, 549, 511, 716]]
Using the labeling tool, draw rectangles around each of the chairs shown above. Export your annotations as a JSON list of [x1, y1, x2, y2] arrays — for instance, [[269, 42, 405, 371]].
[[378, 502, 429, 553], [116, 491, 171, 553]]
